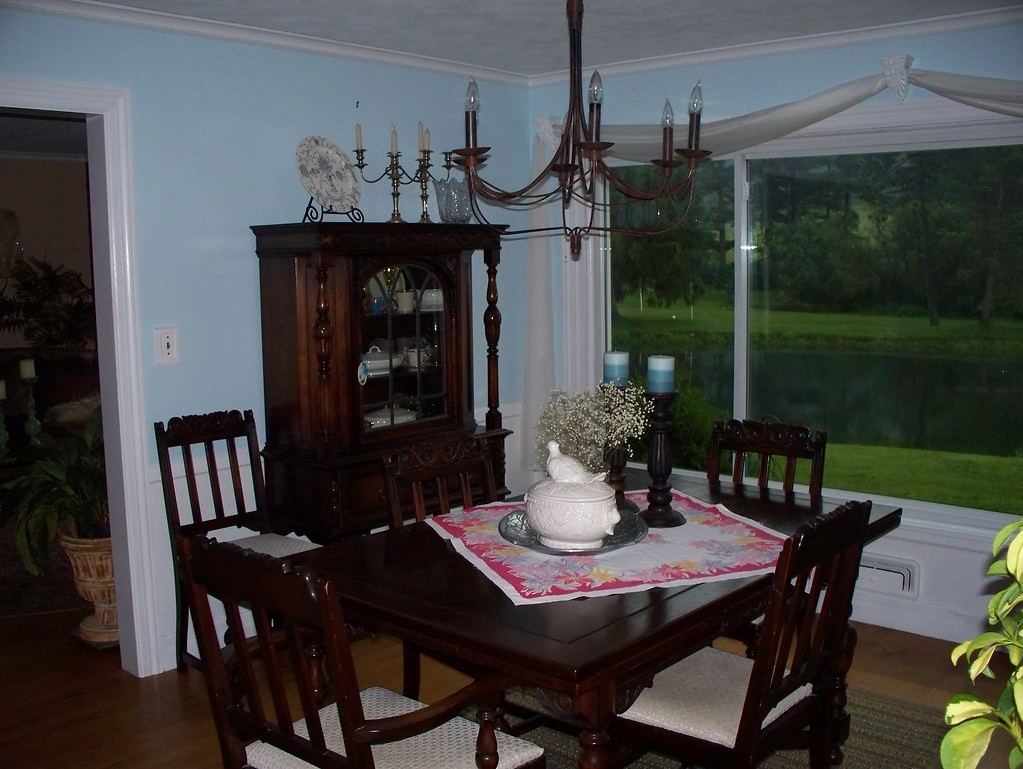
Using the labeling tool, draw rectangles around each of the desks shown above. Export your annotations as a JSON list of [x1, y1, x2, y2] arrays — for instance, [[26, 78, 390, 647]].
[[290, 472, 903, 769]]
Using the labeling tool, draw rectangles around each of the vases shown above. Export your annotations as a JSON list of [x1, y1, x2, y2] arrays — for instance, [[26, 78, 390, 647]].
[[584, 458, 612, 484]]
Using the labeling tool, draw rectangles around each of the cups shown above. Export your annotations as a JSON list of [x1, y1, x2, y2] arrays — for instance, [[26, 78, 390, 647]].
[[395, 290, 415, 313], [406, 348, 425, 371]]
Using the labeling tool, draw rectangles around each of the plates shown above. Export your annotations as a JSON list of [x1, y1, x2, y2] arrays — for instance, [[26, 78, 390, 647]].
[[498, 509, 649, 556], [369, 267, 405, 297], [295, 135, 362, 213]]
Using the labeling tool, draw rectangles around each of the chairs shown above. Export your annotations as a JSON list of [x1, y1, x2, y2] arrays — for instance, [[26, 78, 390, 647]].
[[705, 416, 826, 646], [153, 410, 272, 685], [382, 433, 497, 701], [614, 499, 876, 768], [180, 527, 546, 769]]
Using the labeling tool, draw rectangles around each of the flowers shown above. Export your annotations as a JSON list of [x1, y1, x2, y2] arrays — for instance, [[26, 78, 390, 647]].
[[533, 386, 654, 468]]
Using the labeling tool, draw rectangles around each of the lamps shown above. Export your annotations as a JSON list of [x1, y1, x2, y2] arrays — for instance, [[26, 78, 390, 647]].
[[450, 1, 713, 256]]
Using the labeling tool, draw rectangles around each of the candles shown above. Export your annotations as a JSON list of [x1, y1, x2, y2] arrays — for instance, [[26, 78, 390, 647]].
[[356, 123, 362, 150], [1, 379, 7, 402], [418, 119, 424, 152], [20, 358, 37, 379], [605, 350, 630, 386], [424, 129, 430, 149], [390, 129, 398, 156], [646, 355, 674, 392]]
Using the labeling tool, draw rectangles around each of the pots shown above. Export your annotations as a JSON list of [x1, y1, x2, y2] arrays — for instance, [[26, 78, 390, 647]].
[[361, 346, 404, 376]]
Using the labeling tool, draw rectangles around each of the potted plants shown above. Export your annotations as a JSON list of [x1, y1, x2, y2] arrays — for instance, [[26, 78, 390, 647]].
[[5, 407, 119, 645], [1, 255, 96, 354]]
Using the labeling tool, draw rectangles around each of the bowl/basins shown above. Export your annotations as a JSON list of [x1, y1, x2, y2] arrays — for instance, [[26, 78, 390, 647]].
[[417, 289, 444, 311], [363, 401, 417, 428]]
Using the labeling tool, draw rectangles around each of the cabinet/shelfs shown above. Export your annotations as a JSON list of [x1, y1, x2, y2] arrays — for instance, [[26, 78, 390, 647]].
[[250, 221, 510, 628]]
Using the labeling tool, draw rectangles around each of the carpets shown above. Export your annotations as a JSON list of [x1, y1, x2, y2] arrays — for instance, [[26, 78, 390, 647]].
[[464, 656, 953, 769]]
